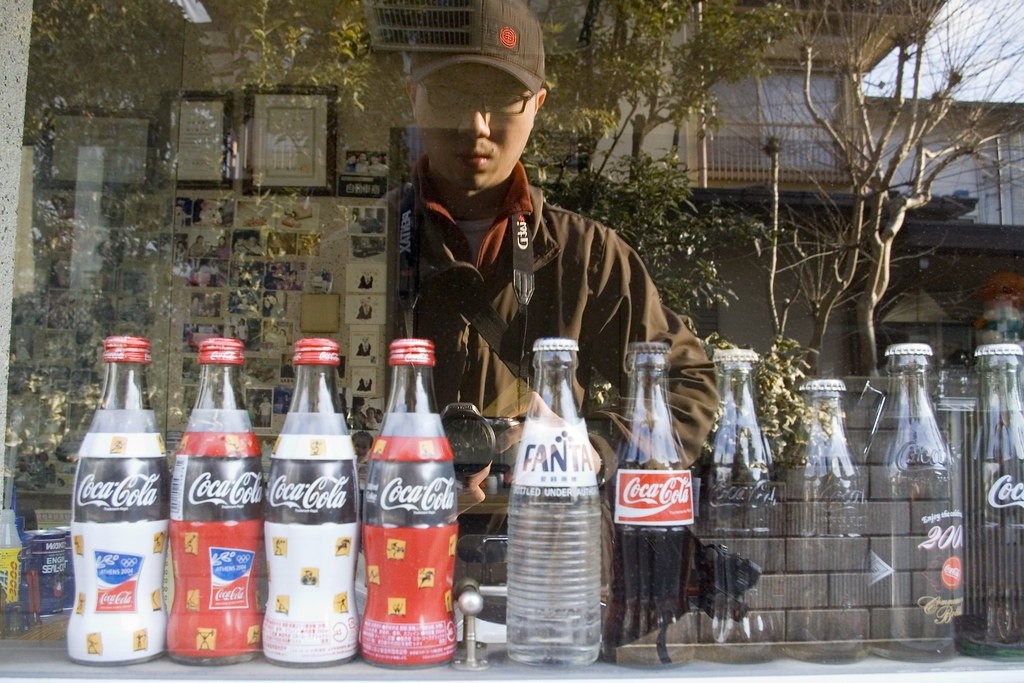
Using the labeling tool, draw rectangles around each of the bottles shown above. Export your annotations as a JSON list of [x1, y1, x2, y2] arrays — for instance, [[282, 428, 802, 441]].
[[691, 349, 782, 664], [68, 336, 170, 666], [261, 338, 362, 668], [361, 338, 459, 670], [864, 342, 955, 663], [167, 335, 262, 664], [782, 379, 864, 665], [508, 337, 600, 667], [955, 346, 1024, 661], [599, 340, 692, 668], [0, 509, 22, 608]]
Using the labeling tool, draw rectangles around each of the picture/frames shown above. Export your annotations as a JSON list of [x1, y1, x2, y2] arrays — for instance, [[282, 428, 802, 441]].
[[243, 83, 337, 197], [40, 105, 154, 196], [160, 88, 236, 192]]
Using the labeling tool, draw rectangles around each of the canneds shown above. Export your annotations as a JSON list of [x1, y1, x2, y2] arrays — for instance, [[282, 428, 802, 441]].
[[21, 525, 76, 618]]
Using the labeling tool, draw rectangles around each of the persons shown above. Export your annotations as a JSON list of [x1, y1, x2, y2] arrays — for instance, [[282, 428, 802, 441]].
[[343, 149, 389, 176], [173, 194, 379, 478], [380, 0, 720, 626]]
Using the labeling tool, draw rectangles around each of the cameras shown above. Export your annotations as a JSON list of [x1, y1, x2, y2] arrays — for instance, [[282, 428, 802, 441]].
[[439, 403, 521, 496]]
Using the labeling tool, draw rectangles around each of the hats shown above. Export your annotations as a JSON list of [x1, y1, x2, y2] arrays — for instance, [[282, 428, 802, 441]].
[[409, 0, 546, 93]]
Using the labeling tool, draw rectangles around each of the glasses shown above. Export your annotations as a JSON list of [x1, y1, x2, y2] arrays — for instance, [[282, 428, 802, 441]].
[[419, 80, 534, 116]]
